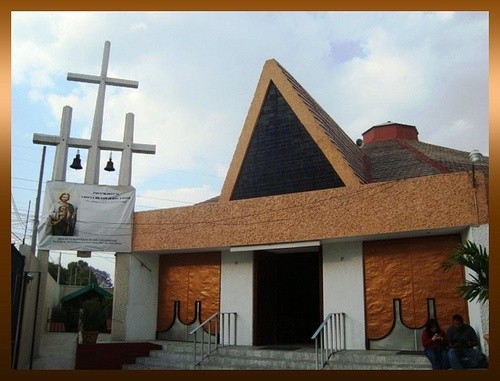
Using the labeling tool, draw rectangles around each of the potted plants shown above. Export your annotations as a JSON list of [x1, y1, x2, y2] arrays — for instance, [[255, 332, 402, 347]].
[[80, 300, 108, 343]]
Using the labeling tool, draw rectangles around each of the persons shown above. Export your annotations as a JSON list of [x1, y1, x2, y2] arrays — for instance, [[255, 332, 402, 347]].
[[47, 192, 79, 237], [446, 314, 487, 369], [422, 317, 452, 368]]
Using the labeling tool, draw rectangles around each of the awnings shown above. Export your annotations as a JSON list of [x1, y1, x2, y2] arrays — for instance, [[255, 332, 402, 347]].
[[59, 282, 113, 334]]
